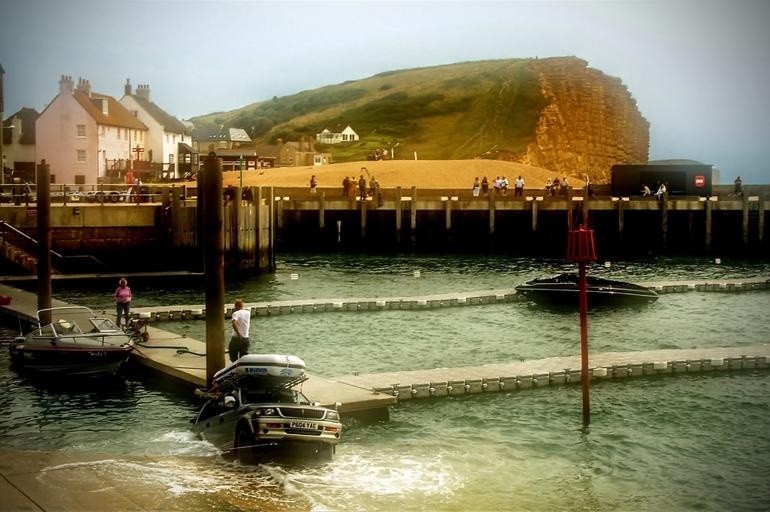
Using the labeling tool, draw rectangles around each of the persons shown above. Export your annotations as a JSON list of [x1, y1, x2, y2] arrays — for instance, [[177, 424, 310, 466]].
[[481, 176, 490, 197], [561, 176, 569, 196], [368, 175, 380, 197], [113, 276, 133, 328], [514, 175, 524, 195], [545, 178, 551, 194], [471, 176, 481, 199], [501, 176, 509, 196], [310, 174, 318, 194], [492, 176, 501, 193], [342, 176, 350, 197], [348, 176, 358, 198], [228, 298, 252, 363], [652, 180, 667, 201], [358, 174, 366, 200], [734, 176, 742, 193], [553, 177, 560, 194], [641, 184, 651, 200]]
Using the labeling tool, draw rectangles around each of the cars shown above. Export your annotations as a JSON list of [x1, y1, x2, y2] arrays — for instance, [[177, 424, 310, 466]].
[[189, 388, 343, 466]]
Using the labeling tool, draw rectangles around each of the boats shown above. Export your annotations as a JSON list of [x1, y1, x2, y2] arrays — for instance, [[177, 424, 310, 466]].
[[9, 306, 136, 383], [515, 272, 659, 306], [212, 353, 306, 386]]
